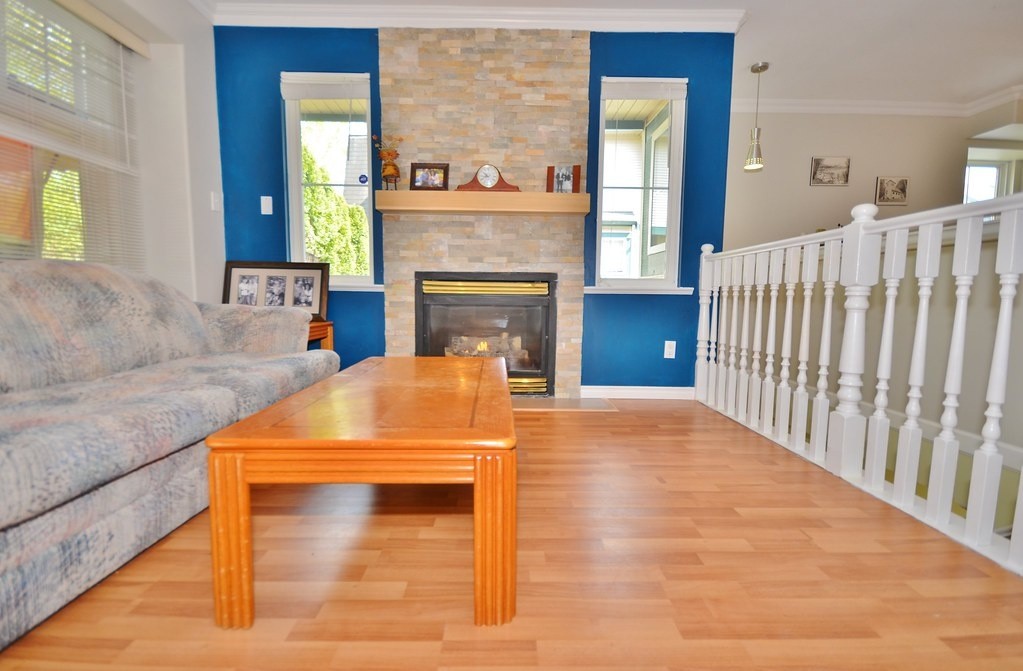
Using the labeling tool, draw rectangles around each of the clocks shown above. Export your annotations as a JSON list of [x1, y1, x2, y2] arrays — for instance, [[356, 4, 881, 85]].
[[456, 164, 521, 192]]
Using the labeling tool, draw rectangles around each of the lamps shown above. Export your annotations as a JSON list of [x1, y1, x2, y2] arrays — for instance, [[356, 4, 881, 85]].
[[744, 62, 769, 170]]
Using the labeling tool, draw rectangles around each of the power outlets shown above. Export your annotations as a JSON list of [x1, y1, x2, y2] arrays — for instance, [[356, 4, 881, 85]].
[[664, 340, 677, 358]]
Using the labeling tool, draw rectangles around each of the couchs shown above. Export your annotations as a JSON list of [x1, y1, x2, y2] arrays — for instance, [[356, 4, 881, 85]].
[[1, 256, 340, 650]]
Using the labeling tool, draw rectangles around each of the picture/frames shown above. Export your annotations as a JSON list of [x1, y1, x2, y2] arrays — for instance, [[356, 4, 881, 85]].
[[222, 260, 330, 322], [409, 163, 449, 190], [546, 164, 581, 193], [874, 177, 908, 206], [809, 156, 851, 186]]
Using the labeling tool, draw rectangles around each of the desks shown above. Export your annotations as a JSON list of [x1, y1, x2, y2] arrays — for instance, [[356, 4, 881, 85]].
[[307, 320, 334, 350]]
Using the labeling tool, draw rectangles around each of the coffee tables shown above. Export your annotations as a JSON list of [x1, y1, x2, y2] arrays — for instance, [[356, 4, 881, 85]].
[[205, 355, 517, 629]]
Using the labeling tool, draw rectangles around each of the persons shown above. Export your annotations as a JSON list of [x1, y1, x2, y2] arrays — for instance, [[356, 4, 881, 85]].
[[556, 168, 572, 193], [238, 276, 258, 306], [271, 281, 280, 306], [419, 169, 439, 186], [297, 283, 313, 306]]
